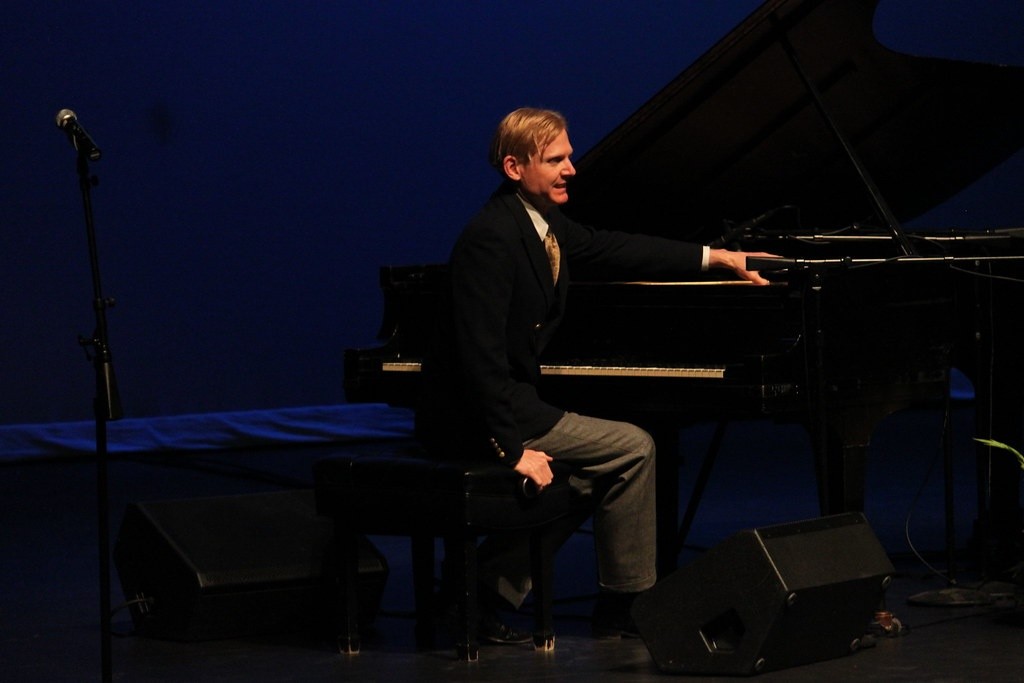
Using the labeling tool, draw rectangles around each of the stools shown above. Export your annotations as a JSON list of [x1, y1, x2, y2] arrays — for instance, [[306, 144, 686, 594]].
[[313, 454, 554, 650]]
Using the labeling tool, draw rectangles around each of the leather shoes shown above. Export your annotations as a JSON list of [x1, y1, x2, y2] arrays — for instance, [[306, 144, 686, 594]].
[[477, 608, 533, 644], [592, 600, 640, 641]]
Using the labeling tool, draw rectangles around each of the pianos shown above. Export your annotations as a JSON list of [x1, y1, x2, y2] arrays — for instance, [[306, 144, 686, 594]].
[[343, 1, 1024, 605]]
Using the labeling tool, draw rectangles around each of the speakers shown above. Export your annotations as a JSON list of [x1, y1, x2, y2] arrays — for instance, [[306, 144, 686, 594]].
[[628, 509, 896, 677], [112, 488, 338, 636]]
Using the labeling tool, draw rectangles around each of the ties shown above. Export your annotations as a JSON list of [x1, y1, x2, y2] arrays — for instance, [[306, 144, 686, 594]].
[[543, 226, 561, 287]]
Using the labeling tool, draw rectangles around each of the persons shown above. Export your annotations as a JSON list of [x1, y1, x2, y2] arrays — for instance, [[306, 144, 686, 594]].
[[413, 107, 784, 640]]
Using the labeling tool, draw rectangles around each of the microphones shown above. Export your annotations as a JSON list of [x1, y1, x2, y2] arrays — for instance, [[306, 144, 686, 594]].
[[56, 109, 101, 160], [705, 209, 776, 249]]
